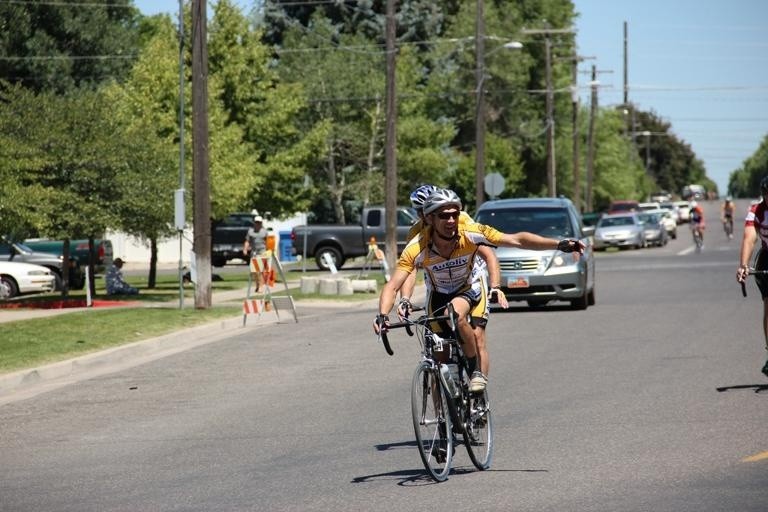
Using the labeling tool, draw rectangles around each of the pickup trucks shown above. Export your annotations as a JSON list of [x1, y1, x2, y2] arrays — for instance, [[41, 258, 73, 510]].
[[292, 205, 421, 271]]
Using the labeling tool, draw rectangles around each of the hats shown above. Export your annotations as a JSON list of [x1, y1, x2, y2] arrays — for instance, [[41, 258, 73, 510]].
[[253, 215, 263, 222], [112, 258, 125, 264]]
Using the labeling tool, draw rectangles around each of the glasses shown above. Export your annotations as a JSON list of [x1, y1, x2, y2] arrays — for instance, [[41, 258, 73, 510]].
[[432, 210, 462, 219]]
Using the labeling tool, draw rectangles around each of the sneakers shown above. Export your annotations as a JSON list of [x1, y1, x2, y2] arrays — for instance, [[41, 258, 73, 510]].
[[468, 370, 488, 393], [431, 440, 456, 457]]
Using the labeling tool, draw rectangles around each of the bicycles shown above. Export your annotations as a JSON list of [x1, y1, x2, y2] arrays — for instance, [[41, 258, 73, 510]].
[[723, 216, 732, 236], [376, 303, 495, 482], [741, 271, 768, 377], [690, 224, 704, 249]]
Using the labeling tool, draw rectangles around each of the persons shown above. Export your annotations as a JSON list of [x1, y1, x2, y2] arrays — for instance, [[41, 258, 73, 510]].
[[395, 183, 511, 422], [242, 215, 267, 294], [372, 187, 586, 456], [104, 258, 138, 296], [720, 196, 735, 238], [687, 201, 704, 242], [736, 177, 768, 377]]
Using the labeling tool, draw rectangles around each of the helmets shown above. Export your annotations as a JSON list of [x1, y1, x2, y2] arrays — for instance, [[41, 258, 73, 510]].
[[690, 201, 698, 209], [409, 184, 440, 211], [422, 189, 463, 217]]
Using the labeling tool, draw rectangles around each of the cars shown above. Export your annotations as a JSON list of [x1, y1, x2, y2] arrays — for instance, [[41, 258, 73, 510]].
[[593, 201, 691, 253]]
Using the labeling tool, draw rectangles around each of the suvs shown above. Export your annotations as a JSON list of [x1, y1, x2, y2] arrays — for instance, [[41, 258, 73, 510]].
[[472, 196, 596, 309], [0, 235, 112, 303], [212, 212, 272, 268]]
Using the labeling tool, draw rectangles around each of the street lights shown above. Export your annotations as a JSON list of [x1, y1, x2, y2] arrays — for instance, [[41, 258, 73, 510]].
[[481, 43, 524, 94], [550, 81, 603, 121], [627, 130, 652, 143]]
